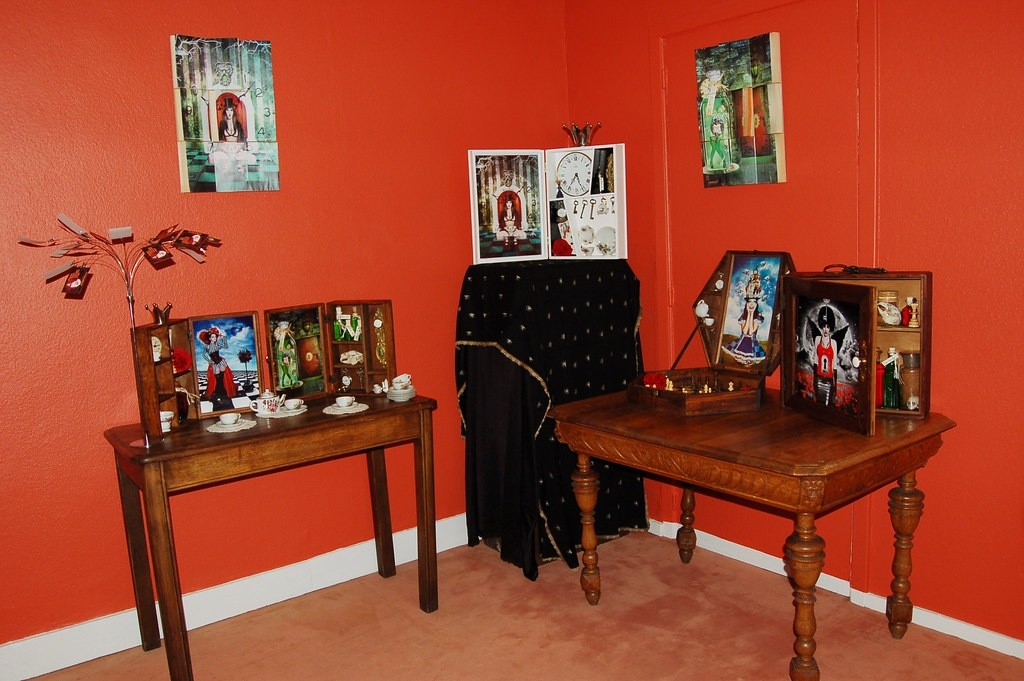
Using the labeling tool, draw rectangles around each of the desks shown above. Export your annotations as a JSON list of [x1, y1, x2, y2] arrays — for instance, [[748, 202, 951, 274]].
[[103, 395, 439, 681], [547, 389, 957, 681]]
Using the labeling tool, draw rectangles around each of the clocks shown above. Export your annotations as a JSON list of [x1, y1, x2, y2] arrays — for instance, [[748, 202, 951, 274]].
[[557, 152, 592, 196]]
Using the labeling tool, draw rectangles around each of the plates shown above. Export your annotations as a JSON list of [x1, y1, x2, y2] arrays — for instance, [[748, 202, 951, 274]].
[[282, 404, 308, 413], [331, 402, 359, 410], [386, 385, 416, 402], [216, 419, 245, 427]]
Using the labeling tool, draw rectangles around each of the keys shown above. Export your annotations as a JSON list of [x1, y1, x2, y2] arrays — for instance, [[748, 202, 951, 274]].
[[572, 199, 596, 220]]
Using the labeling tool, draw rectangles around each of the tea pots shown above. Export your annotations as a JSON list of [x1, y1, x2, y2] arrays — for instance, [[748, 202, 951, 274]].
[[249, 388, 286, 415], [382, 378, 388, 394]]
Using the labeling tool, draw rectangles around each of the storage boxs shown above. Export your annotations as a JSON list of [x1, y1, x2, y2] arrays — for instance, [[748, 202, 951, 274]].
[[129, 302, 266, 438], [624, 249, 798, 417], [782, 267, 932, 436], [264, 300, 397, 407]]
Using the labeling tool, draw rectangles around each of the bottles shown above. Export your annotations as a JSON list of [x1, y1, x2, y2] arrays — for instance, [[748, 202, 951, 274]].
[[875, 346, 919, 408], [275, 321, 299, 387]]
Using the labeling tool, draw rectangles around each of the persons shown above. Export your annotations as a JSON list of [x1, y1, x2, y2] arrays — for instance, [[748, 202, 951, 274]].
[[196, 327, 237, 399], [722, 295, 766, 366], [496, 194, 527, 246], [219, 98, 244, 142], [814, 306, 837, 406]]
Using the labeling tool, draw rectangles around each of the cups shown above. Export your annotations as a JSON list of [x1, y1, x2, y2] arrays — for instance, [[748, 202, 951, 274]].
[[336, 396, 355, 407], [392, 373, 412, 390], [219, 413, 241, 425], [373, 385, 382, 395], [161, 421, 172, 433], [284, 398, 304, 410], [160, 410, 174, 421]]
